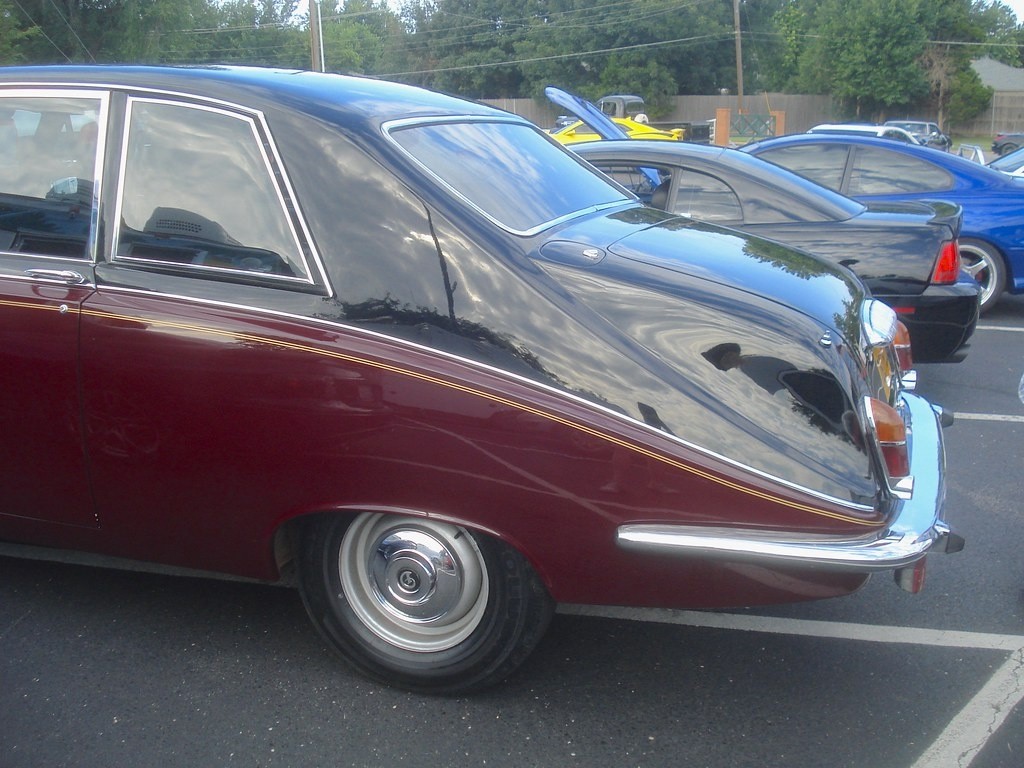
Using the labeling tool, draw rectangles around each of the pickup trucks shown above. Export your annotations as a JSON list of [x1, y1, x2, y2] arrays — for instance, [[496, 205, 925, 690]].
[[554, 95, 710, 144]]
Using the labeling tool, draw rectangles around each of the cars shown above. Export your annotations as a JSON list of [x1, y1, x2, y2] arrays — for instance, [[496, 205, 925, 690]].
[[549, 118, 684, 147], [806, 123, 920, 145], [991, 131, 1024, 155], [882, 121, 953, 153], [985, 146, 1024, 177], [2, 65, 964, 695], [561, 141, 980, 365], [543, 86, 1024, 318]]
